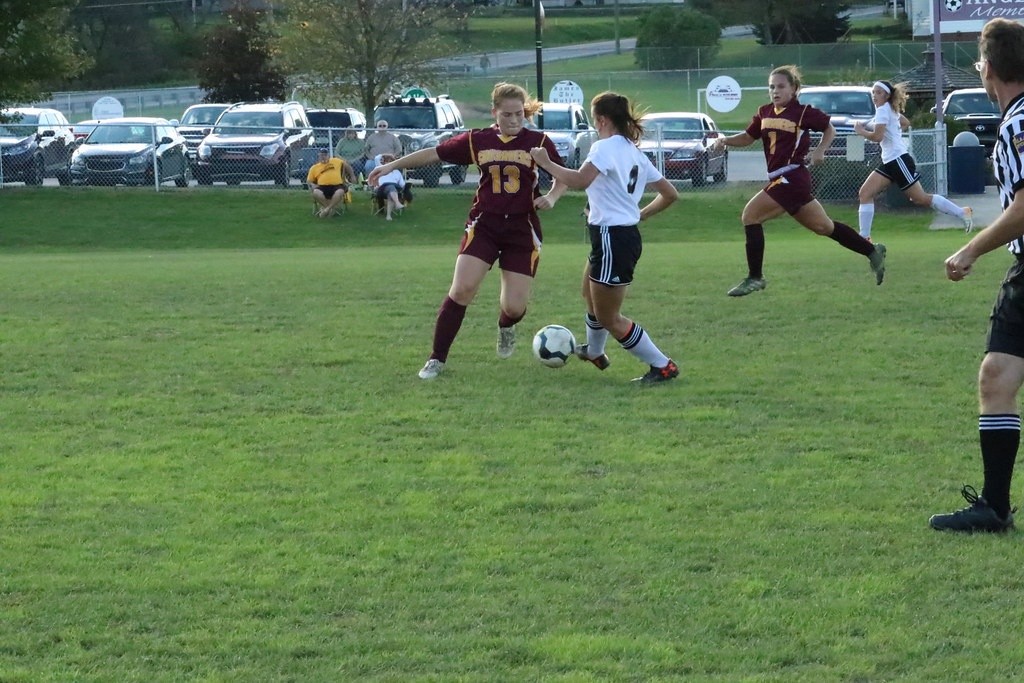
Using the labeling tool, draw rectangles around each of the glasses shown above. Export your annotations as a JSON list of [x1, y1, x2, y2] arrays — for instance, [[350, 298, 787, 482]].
[[379, 124, 386, 127], [975, 62, 985, 71]]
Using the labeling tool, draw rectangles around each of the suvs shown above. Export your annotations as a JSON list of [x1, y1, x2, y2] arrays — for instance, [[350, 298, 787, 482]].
[[170, 103, 234, 179], [196, 100, 316, 188], [524, 103, 597, 170], [0, 108, 76, 186], [305, 94, 469, 187], [930, 88, 1002, 158], [70, 117, 190, 187], [797, 85, 879, 155]]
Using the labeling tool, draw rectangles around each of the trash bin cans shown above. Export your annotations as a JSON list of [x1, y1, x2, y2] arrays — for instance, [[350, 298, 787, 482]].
[[947, 145, 986, 194]]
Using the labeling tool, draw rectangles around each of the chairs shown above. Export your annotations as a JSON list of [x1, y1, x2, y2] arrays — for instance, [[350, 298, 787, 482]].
[[369, 187, 405, 216], [308, 183, 348, 217]]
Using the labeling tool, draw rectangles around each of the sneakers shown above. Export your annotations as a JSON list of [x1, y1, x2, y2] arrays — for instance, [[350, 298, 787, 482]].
[[929, 485, 1013, 532], [419, 359, 445, 379], [497, 320, 515, 359]]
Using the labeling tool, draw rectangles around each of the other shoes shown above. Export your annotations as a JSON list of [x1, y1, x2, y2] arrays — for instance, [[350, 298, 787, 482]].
[[320, 210, 327, 218], [396, 205, 404, 209], [963, 207, 973, 234], [336, 207, 344, 216], [640, 359, 679, 385], [727, 275, 766, 297], [386, 217, 392, 221], [870, 243, 886, 285], [576, 343, 610, 370]]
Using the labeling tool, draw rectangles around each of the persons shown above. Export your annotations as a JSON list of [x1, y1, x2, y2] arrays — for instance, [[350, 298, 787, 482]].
[[364, 120, 402, 177], [929, 20, 1024, 534], [854, 79, 972, 243], [334, 126, 367, 181], [480, 53, 491, 76], [530, 91, 680, 381], [374, 154, 405, 220], [714, 66, 887, 297], [201, 111, 211, 123], [368, 81, 567, 378], [307, 147, 357, 217]]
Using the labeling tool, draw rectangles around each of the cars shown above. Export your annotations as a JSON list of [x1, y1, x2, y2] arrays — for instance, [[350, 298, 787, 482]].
[[636, 112, 728, 187]]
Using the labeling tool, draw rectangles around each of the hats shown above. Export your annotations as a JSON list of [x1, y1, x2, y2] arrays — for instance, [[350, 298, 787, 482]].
[[320, 148, 328, 153], [377, 120, 388, 129]]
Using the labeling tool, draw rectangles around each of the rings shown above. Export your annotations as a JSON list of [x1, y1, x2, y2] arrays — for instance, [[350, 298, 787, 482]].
[[952, 271, 956, 273]]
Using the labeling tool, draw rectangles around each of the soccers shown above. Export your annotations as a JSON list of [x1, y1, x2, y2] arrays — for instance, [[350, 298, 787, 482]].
[[532, 324, 576, 369]]
